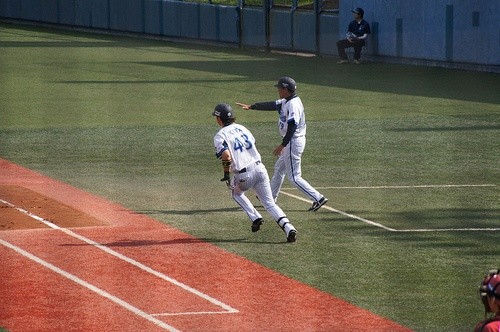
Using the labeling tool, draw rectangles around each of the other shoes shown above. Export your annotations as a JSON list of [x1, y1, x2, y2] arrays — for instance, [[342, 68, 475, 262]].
[[336, 60, 350, 64], [352, 58, 363, 64]]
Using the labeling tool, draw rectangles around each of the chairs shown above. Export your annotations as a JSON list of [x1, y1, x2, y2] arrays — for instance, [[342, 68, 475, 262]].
[[345, 40, 368, 64]]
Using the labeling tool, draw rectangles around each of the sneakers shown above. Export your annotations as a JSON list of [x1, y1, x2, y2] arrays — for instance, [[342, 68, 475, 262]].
[[287, 229, 297, 242], [308, 197, 329, 211], [251, 218, 264, 233]]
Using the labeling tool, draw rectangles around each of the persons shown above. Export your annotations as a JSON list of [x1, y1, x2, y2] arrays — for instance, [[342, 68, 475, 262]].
[[335, 8, 370, 64], [212, 103, 298, 244], [474, 269, 500, 332], [235, 77, 328, 212]]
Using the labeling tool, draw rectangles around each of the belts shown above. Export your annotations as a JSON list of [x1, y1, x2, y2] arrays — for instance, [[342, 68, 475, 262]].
[[239, 160, 261, 174]]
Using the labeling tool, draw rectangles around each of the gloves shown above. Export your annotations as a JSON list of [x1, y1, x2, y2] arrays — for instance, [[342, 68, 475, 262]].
[[224, 179, 236, 191]]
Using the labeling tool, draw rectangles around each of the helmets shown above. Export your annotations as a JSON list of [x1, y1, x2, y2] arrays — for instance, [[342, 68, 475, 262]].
[[211, 103, 235, 120], [481, 274, 500, 296], [273, 77, 297, 92]]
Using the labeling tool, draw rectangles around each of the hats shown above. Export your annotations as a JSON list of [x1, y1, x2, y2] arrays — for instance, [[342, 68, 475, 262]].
[[351, 7, 365, 14]]
